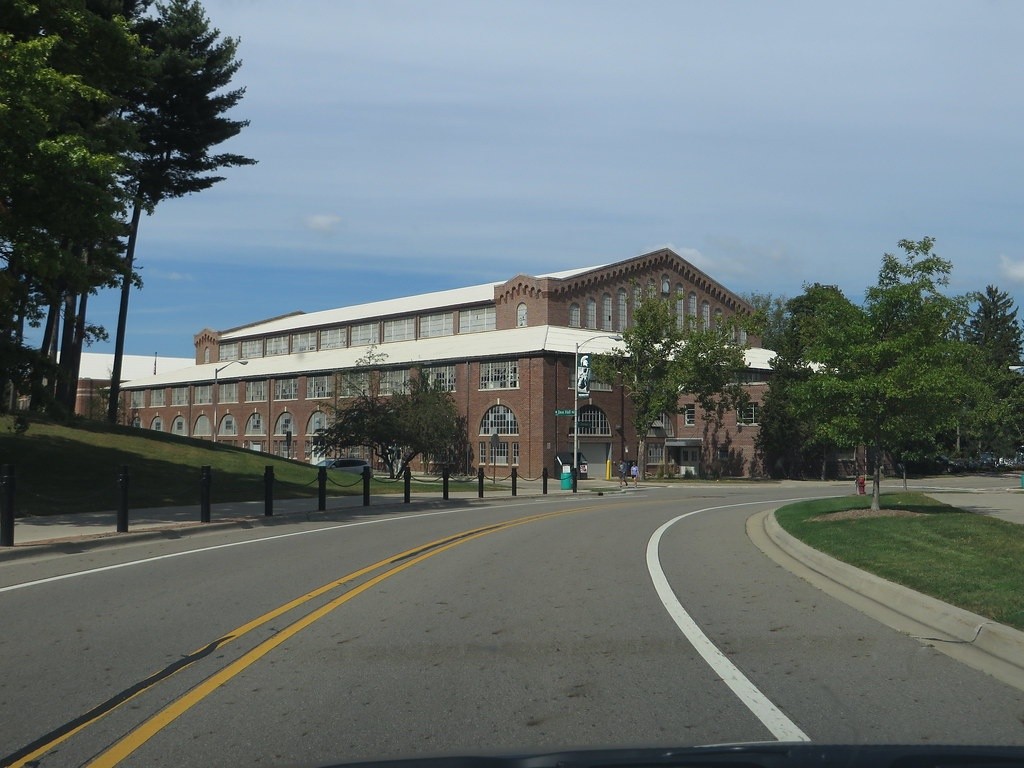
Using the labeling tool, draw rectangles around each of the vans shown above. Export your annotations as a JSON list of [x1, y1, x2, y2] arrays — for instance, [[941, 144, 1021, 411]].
[[317, 458, 374, 478]]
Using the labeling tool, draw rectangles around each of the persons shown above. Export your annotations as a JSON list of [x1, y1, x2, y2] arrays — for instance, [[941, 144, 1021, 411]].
[[617, 459, 628, 488], [579, 369, 590, 392], [631, 462, 639, 487]]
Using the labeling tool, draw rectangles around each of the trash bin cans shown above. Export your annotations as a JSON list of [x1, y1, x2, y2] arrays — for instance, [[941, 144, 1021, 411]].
[[561, 473, 573, 490], [1021, 473, 1024, 489], [554, 452, 588, 480]]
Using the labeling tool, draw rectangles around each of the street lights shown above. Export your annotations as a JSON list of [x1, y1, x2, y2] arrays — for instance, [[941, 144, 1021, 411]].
[[573, 335, 623, 493], [214, 360, 248, 443]]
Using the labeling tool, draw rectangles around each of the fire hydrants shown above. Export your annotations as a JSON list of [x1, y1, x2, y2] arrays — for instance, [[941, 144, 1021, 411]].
[[857, 477, 865, 494]]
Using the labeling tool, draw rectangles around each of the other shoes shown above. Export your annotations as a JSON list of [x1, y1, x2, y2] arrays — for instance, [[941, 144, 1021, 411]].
[[619, 485, 622, 488], [626, 483, 628, 486]]
